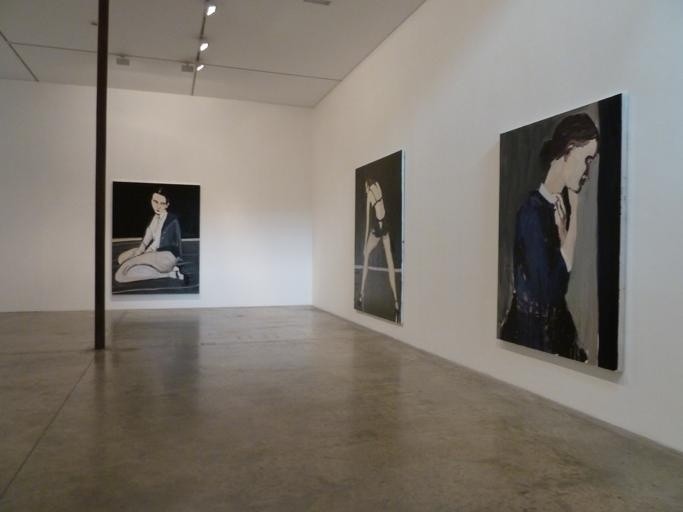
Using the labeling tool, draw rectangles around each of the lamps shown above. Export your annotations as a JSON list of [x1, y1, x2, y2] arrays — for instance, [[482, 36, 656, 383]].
[[193, 0, 217, 72], [115, 52, 194, 74]]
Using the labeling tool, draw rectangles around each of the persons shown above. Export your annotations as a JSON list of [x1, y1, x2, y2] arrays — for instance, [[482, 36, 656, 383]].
[[116, 187, 190, 285], [358, 175, 400, 324], [508, 113, 599, 363]]
